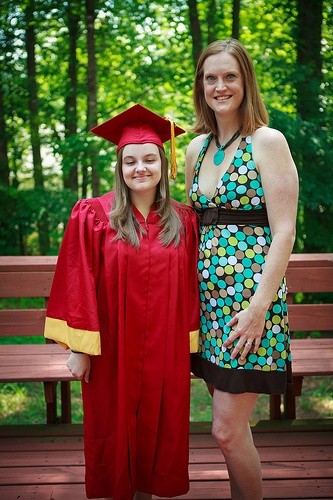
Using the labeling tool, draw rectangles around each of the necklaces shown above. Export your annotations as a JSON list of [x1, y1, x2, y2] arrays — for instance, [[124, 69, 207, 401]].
[[213, 127, 241, 166]]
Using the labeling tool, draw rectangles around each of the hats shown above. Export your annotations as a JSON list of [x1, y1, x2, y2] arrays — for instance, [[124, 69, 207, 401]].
[[90, 104, 186, 180]]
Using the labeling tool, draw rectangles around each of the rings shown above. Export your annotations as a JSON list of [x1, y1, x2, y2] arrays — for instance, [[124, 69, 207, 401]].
[[247, 341, 252, 346]]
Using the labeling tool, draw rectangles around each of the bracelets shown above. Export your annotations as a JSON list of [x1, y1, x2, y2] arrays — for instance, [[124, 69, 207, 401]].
[[71, 350, 87, 355]]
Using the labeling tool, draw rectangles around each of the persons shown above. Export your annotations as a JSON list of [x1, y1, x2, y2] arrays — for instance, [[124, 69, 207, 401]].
[[43, 105, 202, 500], [184, 40, 299, 500]]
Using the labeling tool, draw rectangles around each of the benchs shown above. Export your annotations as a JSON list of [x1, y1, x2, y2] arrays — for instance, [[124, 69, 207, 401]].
[[0, 253, 333, 424]]
